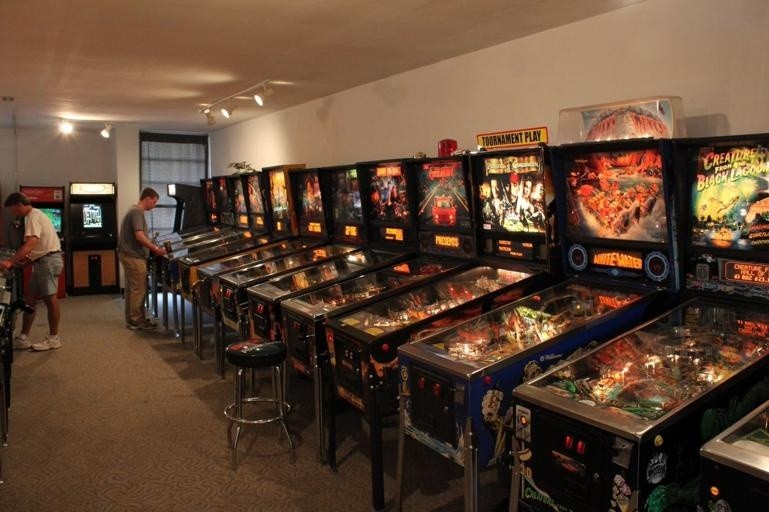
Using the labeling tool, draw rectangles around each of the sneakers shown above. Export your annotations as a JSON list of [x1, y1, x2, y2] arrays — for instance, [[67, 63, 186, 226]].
[[30, 335, 61, 350], [13, 337, 32, 349], [130, 322, 157, 330]]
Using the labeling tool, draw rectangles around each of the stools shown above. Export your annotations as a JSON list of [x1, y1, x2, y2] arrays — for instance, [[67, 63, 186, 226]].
[[222, 339, 298, 472]]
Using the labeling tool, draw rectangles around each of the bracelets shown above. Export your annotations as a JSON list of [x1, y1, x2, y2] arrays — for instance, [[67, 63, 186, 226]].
[[152, 246, 158, 251]]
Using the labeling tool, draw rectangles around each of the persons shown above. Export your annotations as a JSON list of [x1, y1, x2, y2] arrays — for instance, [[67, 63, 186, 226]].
[[0, 192, 64, 352], [116, 186, 168, 331]]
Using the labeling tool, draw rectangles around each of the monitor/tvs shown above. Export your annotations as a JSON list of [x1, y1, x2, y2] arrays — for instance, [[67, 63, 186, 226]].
[[298, 173, 324, 218], [218, 179, 228, 208], [81, 204, 105, 229], [366, 165, 409, 221], [479, 154, 545, 236], [416, 161, 472, 227], [690, 144, 769, 251], [329, 169, 362, 222], [233, 179, 247, 214], [564, 150, 670, 245], [206, 181, 217, 209], [36, 208, 62, 234], [247, 176, 264, 214], [267, 170, 288, 218]]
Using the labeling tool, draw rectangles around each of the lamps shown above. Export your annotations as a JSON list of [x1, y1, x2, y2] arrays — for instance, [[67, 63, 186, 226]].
[[197, 78, 276, 127], [100, 120, 114, 139]]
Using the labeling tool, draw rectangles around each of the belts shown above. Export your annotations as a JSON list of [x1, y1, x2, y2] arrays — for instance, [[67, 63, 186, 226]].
[[33, 251, 58, 261]]
